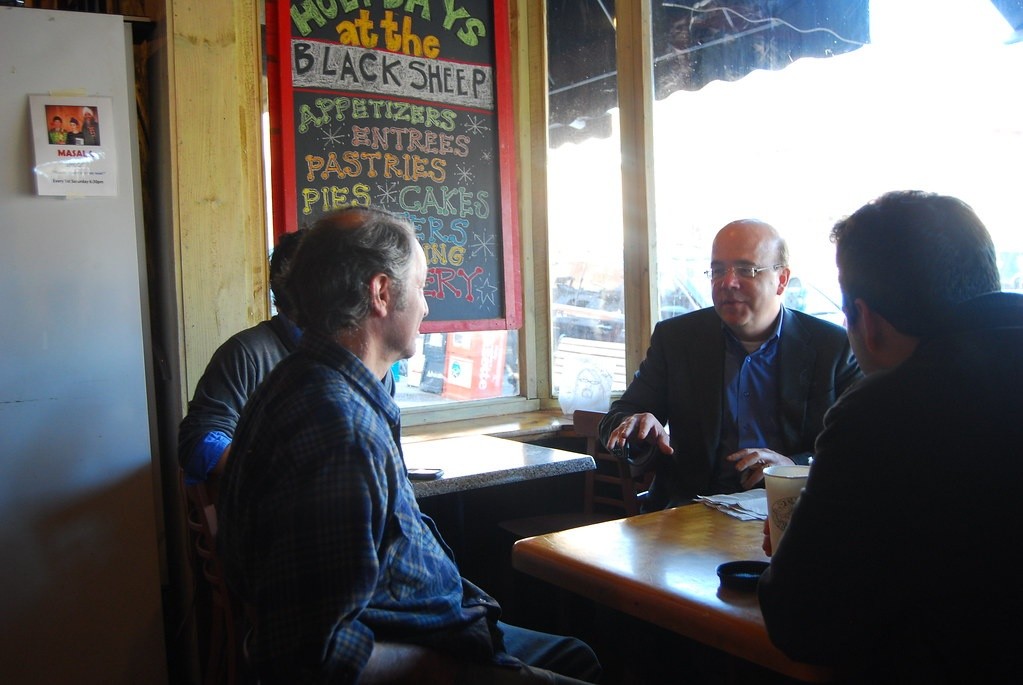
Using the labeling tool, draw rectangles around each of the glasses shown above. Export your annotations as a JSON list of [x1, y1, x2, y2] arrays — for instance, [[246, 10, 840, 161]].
[[704, 263, 783, 280]]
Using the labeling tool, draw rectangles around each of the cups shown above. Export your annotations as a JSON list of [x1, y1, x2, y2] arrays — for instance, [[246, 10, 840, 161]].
[[763, 464, 812, 557]]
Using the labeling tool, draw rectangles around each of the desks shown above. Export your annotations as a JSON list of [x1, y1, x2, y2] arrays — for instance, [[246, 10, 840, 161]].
[[514, 504, 811, 685], [402, 434, 596, 499]]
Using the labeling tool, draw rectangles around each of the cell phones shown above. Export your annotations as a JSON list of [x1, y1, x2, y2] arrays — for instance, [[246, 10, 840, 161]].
[[407, 469, 444, 481]]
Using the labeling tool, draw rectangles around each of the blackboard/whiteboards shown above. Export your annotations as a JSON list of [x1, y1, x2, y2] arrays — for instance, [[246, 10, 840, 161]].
[[266, 0, 524, 333]]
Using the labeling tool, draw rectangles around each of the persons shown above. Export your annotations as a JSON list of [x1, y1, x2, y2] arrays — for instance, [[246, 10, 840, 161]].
[[215, 204, 597, 685], [48, 107, 100, 146], [756, 198, 1023, 685], [599, 218, 863, 513], [182, 228, 599, 685]]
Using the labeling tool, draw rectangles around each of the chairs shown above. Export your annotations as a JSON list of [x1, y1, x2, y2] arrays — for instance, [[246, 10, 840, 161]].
[[494, 410, 626, 605]]
